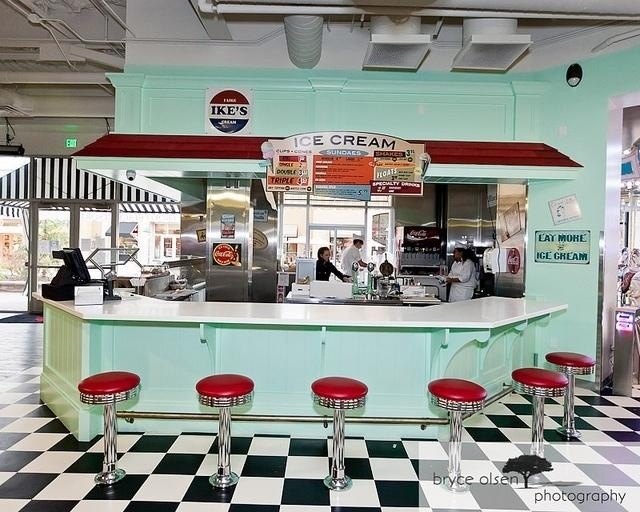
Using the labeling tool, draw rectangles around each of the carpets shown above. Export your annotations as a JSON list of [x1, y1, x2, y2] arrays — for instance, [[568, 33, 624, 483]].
[[0, 311, 45, 324]]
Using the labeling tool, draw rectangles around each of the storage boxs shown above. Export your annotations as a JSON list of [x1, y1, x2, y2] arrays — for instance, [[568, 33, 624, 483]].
[[399, 285, 427, 300], [309, 279, 353, 299], [291, 282, 311, 296]]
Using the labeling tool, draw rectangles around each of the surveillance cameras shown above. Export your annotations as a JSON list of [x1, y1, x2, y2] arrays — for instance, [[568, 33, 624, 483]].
[[126, 171, 136, 180]]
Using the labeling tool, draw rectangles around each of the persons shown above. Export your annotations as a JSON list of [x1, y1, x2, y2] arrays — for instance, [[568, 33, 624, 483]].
[[316, 247, 354, 283], [340, 238, 368, 281], [435, 243, 480, 303]]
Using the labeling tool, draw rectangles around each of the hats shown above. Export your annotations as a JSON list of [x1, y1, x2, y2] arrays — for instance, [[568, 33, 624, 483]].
[[454, 239, 469, 251], [352, 232, 366, 244]]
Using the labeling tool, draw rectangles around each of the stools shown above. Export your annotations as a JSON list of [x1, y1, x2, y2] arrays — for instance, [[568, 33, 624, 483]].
[[195, 374, 256, 486], [510, 366, 570, 461], [77, 369, 142, 485], [310, 376, 369, 490], [424, 376, 487, 491], [544, 349, 597, 439]]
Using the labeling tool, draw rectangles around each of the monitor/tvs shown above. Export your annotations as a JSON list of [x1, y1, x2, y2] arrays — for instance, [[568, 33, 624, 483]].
[[62, 248, 90, 281]]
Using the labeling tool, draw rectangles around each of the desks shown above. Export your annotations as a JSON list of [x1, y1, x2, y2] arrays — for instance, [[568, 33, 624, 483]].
[[285, 282, 442, 305]]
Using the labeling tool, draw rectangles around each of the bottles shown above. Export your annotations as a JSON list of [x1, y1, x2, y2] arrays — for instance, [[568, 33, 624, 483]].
[[511, 249, 516, 273], [404, 279, 421, 286]]
